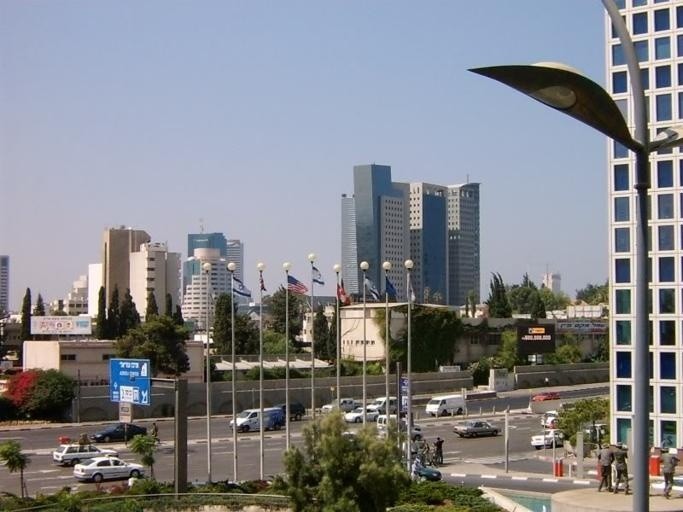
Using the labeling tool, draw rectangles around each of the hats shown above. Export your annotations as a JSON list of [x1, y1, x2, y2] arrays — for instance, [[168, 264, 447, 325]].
[[616, 441, 624, 446], [601, 443, 610, 449], [660, 447, 669, 452]]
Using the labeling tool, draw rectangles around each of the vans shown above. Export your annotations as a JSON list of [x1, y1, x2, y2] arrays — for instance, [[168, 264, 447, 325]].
[[425, 394, 464, 417], [229, 407, 284, 433], [275, 402, 305, 421]]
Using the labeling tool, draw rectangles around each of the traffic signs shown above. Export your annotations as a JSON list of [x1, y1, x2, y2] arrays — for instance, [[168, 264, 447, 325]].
[[109, 357, 151, 406]]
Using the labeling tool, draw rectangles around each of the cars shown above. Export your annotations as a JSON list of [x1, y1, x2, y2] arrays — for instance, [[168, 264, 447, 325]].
[[53, 443, 118, 467], [73, 456, 145, 484], [529, 402, 609, 450], [453, 420, 501, 439], [89, 422, 147, 444], [324, 396, 423, 454], [532, 391, 561, 403]]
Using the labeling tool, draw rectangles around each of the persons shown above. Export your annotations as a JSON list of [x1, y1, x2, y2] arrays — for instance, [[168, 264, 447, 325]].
[[656, 448, 680, 498], [411, 437, 445, 467], [149, 422, 161, 444], [612, 444, 630, 495], [597, 444, 615, 492]]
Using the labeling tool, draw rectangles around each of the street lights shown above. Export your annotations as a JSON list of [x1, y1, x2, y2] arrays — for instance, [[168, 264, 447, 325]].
[[225, 261, 237, 484], [381, 261, 391, 434], [465, 0, 683, 511], [307, 253, 318, 419], [333, 263, 342, 410], [202, 262, 213, 482], [282, 262, 291, 451], [359, 261, 370, 431], [402, 259, 415, 475], [256, 262, 266, 482]]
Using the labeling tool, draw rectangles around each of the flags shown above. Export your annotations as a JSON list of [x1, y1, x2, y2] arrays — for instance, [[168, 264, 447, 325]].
[[287, 276, 307, 297], [337, 274, 350, 304], [364, 274, 379, 301], [385, 274, 397, 298], [258, 271, 267, 291], [232, 276, 251, 298], [310, 265, 324, 287], [207, 274, 216, 306], [407, 275, 415, 312]]
